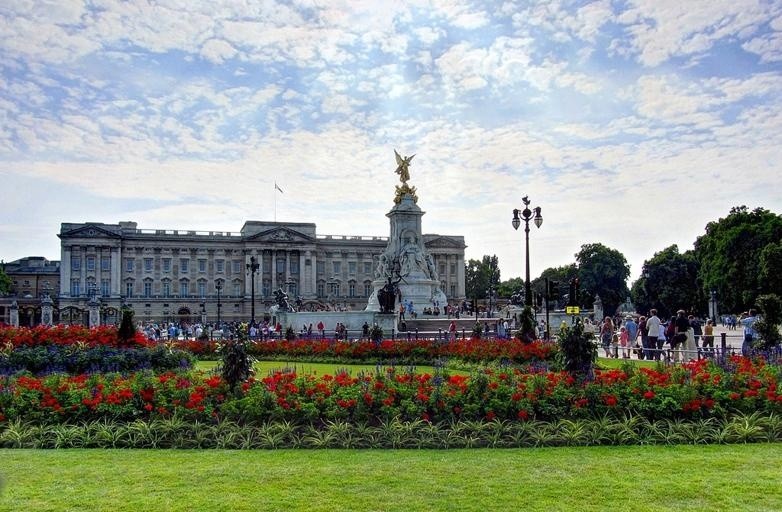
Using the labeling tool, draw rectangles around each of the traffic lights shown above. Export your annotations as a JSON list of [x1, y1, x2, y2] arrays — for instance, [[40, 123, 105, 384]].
[[468, 299, 475, 312], [549, 281, 559, 301]]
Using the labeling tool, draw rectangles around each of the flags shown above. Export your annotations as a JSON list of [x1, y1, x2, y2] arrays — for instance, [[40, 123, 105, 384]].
[[276, 183, 284, 193]]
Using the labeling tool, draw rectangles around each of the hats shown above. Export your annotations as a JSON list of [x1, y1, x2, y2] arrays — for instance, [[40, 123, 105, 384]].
[[624, 315, 633, 321], [676, 309, 685, 314]]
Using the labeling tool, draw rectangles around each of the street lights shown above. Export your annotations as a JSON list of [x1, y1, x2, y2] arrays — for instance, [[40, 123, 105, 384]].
[[512, 196, 543, 306], [709, 288, 718, 326], [246, 256, 259, 321], [216, 280, 222, 330]]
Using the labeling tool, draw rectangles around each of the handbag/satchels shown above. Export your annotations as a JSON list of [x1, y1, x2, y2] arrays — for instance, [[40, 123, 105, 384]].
[[673, 334, 687, 344]]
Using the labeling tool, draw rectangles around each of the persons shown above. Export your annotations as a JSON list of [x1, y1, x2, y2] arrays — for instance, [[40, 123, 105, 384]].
[[301, 320, 370, 338], [295, 296, 303, 312], [135, 319, 282, 340], [531, 308, 762, 363], [395, 298, 520, 342], [379, 274, 401, 313]]
